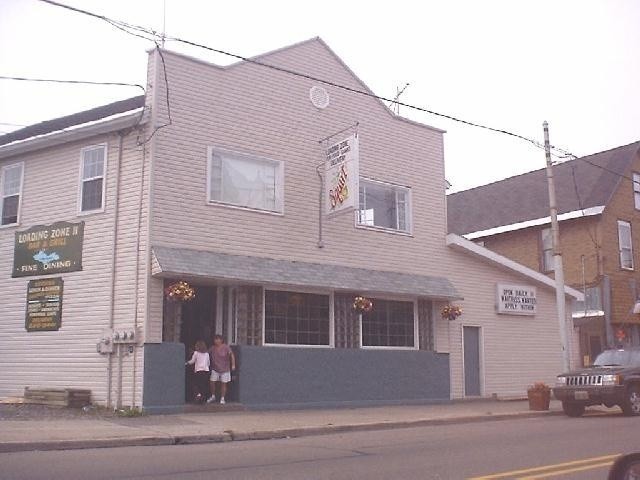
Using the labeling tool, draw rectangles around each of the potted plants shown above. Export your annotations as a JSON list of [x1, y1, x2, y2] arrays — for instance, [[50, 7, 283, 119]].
[[526, 380, 551, 411]]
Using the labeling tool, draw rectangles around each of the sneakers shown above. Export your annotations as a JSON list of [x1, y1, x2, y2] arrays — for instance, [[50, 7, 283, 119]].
[[219, 397, 226, 405], [206, 396, 217, 404]]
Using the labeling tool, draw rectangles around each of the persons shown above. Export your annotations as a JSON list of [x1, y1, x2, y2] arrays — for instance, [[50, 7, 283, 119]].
[[185, 338, 211, 403], [207, 334, 235, 405]]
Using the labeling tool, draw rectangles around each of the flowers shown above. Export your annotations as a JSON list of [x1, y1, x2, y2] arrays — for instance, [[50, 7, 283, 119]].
[[351, 295, 373, 316], [165, 280, 196, 302], [440, 305, 463, 320]]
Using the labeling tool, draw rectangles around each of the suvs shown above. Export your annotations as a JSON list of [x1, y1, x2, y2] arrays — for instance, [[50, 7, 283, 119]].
[[551, 345, 639, 419]]
